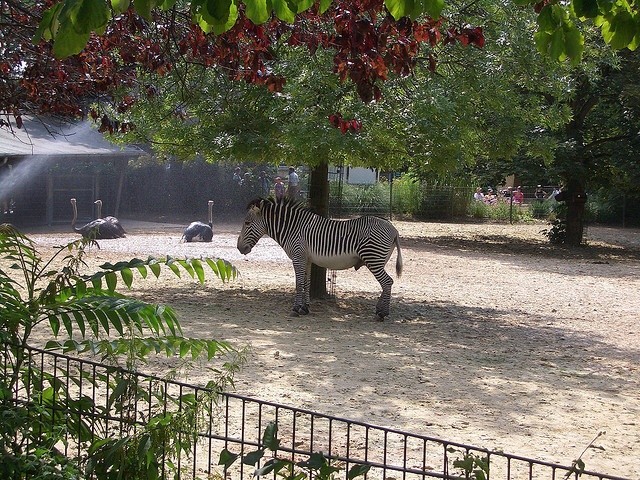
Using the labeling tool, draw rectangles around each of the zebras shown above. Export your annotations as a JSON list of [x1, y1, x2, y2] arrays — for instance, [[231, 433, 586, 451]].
[[236, 193, 404, 323]]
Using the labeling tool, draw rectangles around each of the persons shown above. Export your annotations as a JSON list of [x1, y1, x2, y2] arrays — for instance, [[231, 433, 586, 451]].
[[286, 166, 300, 194], [233, 166, 241, 183], [241, 173, 253, 186], [552, 187, 557, 194], [258, 171, 270, 197], [535, 184, 547, 199], [512, 186, 523, 204], [275, 177, 285, 198], [474, 186, 484, 205], [485, 189, 497, 206], [559, 184, 564, 192]]
